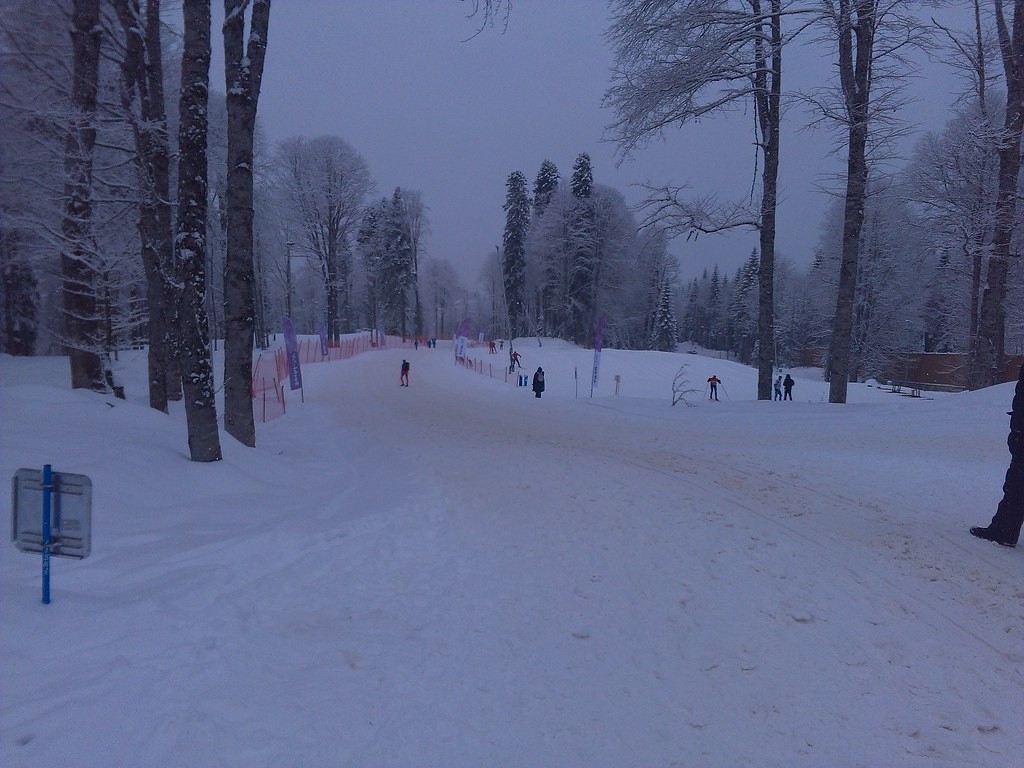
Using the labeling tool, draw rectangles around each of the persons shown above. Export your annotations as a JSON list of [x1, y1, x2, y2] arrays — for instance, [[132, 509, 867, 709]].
[[489, 340, 522, 369], [415, 338, 437, 350], [533, 366, 545, 399], [783, 374, 794, 401], [400, 360, 410, 387], [774, 376, 782, 401], [969, 361, 1024, 549], [707, 374, 722, 401]]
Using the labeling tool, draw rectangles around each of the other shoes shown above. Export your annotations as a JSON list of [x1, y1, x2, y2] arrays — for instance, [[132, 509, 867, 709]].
[[401, 384, 404, 386], [405, 385, 408, 387], [971, 528, 1015, 548]]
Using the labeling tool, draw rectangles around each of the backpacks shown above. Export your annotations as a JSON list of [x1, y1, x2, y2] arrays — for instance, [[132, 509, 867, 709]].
[[537, 372, 544, 381]]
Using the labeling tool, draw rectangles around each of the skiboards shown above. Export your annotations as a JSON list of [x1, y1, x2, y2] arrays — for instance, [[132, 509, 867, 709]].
[[708, 399, 722, 402]]
